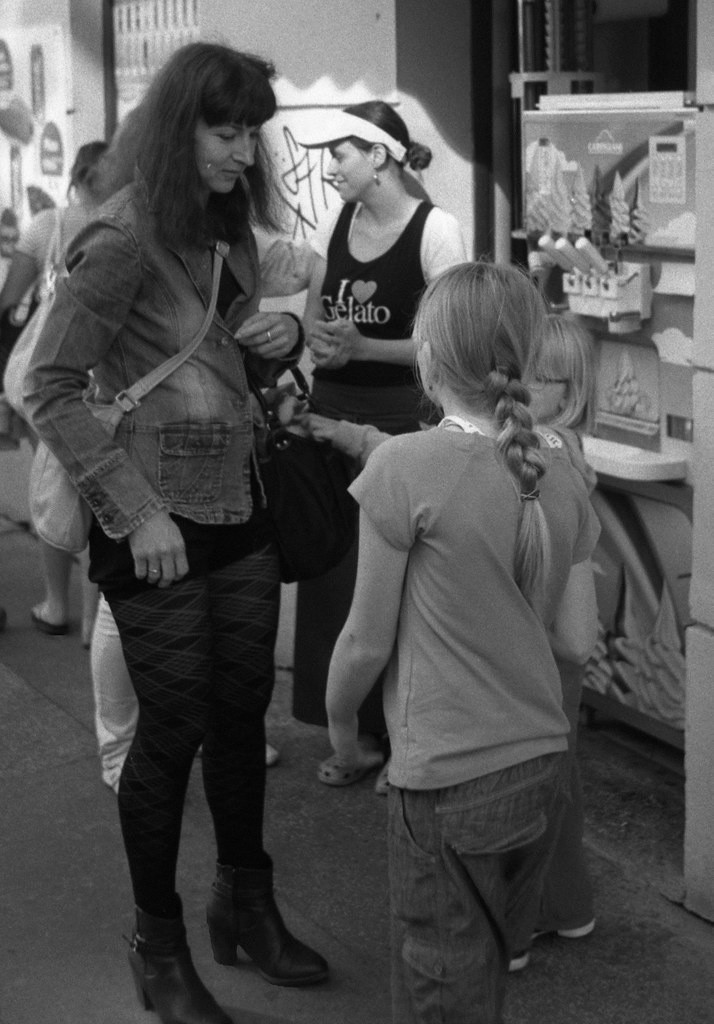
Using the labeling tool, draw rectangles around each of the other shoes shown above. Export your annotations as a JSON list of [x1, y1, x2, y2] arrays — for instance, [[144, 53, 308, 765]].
[[265, 740, 280, 766]]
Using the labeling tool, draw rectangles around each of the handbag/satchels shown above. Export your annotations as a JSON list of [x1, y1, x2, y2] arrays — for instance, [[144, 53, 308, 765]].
[[28, 401, 125, 553], [243, 361, 352, 583], [4, 208, 59, 434]]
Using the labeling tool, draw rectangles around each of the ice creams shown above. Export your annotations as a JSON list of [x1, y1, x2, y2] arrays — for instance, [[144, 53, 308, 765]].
[[525, 139, 647, 248]]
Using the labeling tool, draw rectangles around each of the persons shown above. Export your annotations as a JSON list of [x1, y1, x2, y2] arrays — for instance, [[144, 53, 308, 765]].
[[20, 43, 334, 1023], [290, 97, 467, 798], [0, 138, 116, 649], [325, 268, 597, 1015]]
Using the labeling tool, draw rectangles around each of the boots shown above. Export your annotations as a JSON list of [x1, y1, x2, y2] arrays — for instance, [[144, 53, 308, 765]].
[[210, 848, 328, 984], [132, 900, 231, 1023]]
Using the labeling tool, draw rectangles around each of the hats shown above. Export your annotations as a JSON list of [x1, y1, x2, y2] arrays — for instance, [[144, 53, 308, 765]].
[[290, 112, 411, 167]]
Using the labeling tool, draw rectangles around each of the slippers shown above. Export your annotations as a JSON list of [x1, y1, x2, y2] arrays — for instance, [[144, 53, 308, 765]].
[[315, 744, 385, 785], [31, 601, 68, 632]]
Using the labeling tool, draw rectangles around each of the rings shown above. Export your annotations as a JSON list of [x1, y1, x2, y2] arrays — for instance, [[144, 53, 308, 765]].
[[263, 318, 273, 328], [268, 329, 272, 342], [149, 568, 160, 574]]
